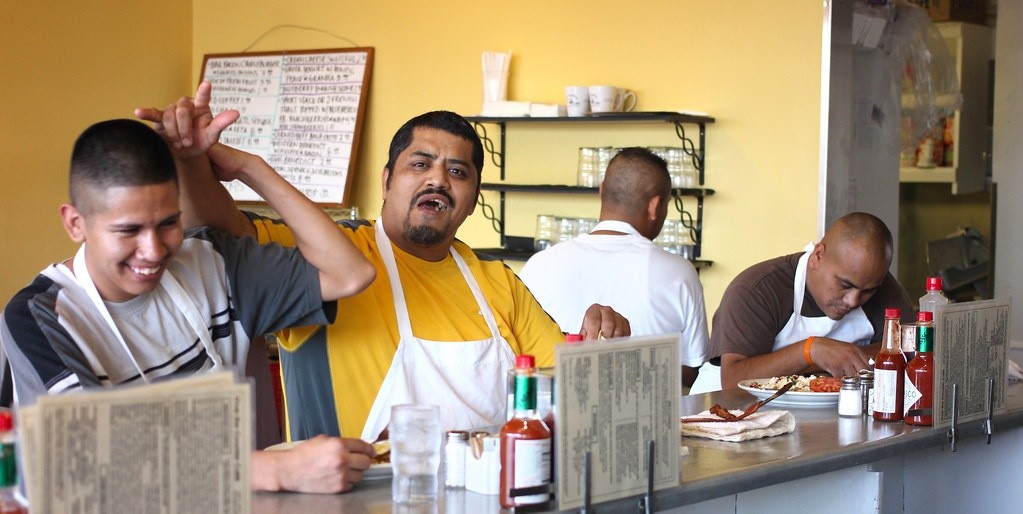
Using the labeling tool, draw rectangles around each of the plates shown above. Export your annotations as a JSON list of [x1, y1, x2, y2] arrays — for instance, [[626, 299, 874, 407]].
[[737, 377, 840, 408]]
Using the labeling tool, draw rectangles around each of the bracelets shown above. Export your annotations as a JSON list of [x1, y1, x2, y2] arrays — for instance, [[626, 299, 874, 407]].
[[804, 335, 817, 370]]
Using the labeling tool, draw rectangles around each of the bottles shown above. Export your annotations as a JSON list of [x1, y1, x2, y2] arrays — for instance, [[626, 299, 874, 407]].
[[871, 307, 908, 423], [918, 277, 949, 322], [0, 411, 30, 514], [836, 370, 875, 416], [496, 334, 584, 514], [443, 429, 492, 491], [903, 311, 935, 426]]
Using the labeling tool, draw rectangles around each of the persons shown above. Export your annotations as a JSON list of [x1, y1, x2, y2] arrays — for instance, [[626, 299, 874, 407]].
[[710, 211, 919, 392], [132, 80, 631, 469], [0, 118, 379, 506], [518, 148, 708, 396]]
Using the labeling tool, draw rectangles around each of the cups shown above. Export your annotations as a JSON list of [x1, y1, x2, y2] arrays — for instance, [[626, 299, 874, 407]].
[[564, 84, 637, 117], [574, 144, 701, 189], [387, 402, 443, 505], [533, 212, 698, 263], [483, 71, 507, 102]]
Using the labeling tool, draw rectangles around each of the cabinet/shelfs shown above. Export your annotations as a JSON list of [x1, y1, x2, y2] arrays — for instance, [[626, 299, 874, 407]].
[[461, 110, 716, 274], [894, 20, 991, 192]]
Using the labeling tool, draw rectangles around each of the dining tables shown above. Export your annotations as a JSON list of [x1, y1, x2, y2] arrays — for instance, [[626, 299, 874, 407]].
[[249, 370, 1016, 514]]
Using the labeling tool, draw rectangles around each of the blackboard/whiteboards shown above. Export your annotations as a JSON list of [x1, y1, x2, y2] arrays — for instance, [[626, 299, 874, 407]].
[[197, 47, 375, 208]]
